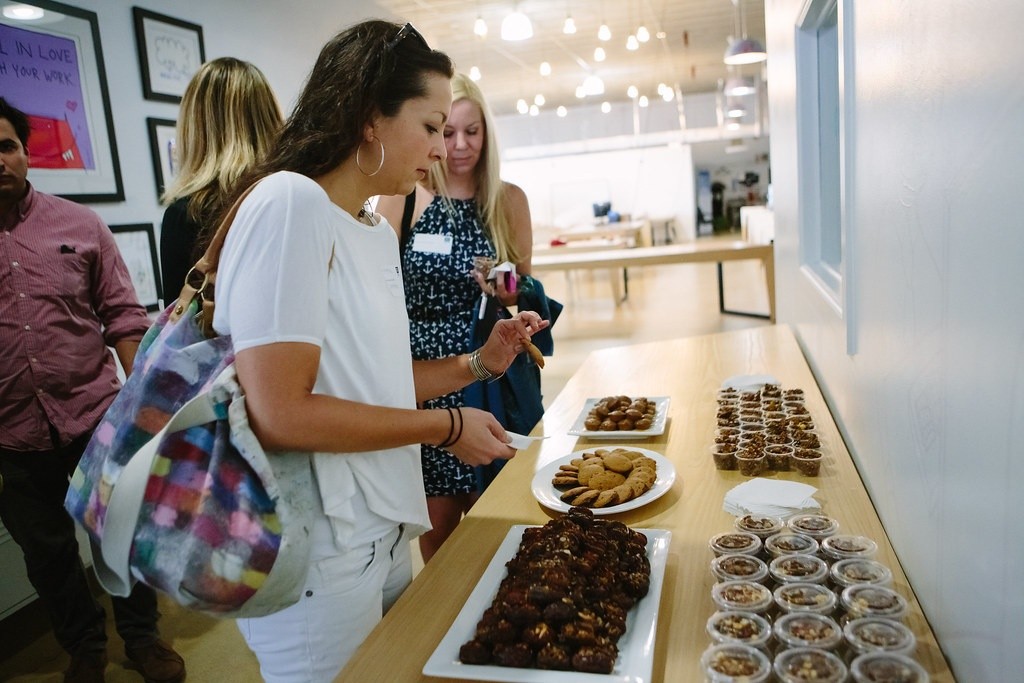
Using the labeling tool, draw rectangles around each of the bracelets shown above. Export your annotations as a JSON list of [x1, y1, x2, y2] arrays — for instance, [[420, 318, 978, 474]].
[[431, 405, 463, 448], [469, 349, 505, 385]]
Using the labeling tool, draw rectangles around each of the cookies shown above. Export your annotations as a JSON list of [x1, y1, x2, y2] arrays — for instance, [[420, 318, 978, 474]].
[[518, 336, 545, 369], [552, 448, 658, 507]]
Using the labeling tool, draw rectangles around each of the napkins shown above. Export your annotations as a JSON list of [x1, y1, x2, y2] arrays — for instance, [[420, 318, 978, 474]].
[[723, 476, 822, 525], [721, 362, 782, 391], [506, 430, 550, 450]]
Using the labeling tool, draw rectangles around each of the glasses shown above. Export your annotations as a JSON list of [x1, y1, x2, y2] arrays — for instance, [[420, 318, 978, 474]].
[[367, 22, 430, 71]]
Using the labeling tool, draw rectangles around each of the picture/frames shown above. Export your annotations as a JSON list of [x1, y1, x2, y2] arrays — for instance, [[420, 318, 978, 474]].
[[130, 4, 208, 104], [0, 0, 127, 205], [107, 222, 164, 314], [146, 117, 176, 206]]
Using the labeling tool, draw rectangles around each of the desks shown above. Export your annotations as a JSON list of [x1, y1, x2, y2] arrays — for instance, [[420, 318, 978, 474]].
[[333, 322, 956, 683], [529, 220, 776, 323]]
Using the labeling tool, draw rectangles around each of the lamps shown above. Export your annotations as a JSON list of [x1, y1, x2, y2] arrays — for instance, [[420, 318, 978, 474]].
[[473, 0, 488, 36], [501, 0, 534, 41], [562, 1, 649, 63], [722, 0, 767, 129]]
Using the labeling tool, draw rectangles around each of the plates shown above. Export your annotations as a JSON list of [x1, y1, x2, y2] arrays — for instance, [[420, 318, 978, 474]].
[[568, 396, 669, 440], [531, 447, 676, 515], [422, 525, 671, 682]]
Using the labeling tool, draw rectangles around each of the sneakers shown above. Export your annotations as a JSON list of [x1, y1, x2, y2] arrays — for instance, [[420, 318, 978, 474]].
[[62, 643, 110, 683], [124, 637, 186, 682]]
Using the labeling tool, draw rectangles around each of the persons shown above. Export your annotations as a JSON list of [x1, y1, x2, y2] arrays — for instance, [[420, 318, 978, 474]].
[[0, 98, 186, 683], [373, 72, 546, 561], [212, 22, 434, 683], [162, 58, 288, 309]]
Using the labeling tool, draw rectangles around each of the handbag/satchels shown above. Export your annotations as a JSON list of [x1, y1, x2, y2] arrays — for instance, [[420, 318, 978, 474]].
[[62, 179, 314, 617]]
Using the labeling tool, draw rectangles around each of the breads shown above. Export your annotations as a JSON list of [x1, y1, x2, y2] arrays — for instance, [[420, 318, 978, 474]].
[[584, 396, 657, 431]]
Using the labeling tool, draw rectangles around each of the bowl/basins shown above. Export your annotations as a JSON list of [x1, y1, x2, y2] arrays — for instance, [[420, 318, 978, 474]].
[[699, 514, 931, 682], [792, 449, 823, 477], [733, 450, 766, 477], [712, 443, 739, 471], [764, 444, 794, 471], [710, 387, 820, 450]]
